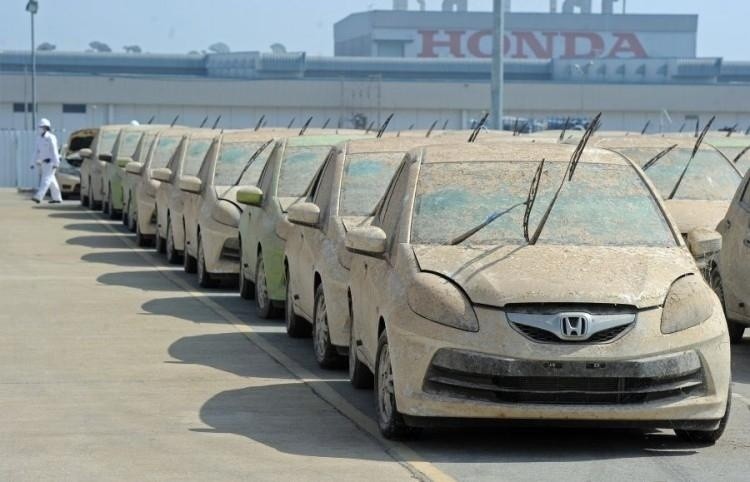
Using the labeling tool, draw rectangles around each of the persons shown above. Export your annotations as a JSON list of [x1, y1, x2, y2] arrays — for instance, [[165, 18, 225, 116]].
[[29, 117, 64, 204]]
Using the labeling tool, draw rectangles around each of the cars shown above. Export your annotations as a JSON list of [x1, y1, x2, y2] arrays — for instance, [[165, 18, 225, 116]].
[[180, 131, 312, 288], [236, 136, 367, 317], [53, 116, 750, 248], [281, 143, 417, 367], [150, 132, 227, 262], [706, 165, 750, 342], [343, 141, 733, 453]]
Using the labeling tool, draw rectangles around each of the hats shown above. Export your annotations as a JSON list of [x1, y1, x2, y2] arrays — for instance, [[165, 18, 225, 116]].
[[40, 119, 50, 127]]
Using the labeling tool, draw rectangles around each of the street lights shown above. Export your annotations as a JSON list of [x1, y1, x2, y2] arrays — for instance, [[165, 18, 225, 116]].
[[23, 0, 42, 130]]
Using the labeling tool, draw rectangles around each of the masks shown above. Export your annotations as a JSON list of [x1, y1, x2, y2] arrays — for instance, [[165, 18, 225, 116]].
[[39, 128, 44, 135]]
